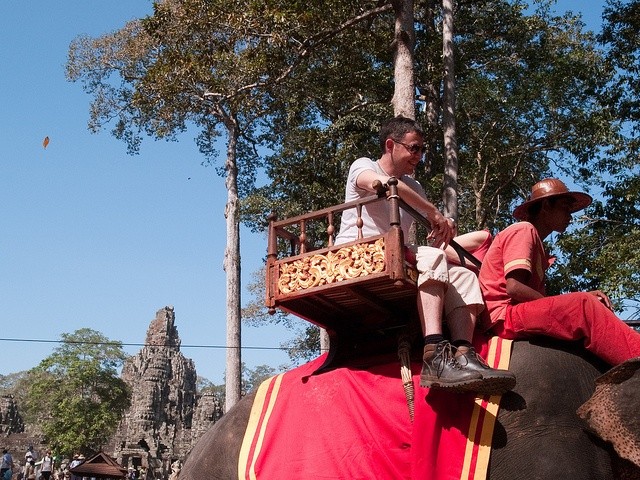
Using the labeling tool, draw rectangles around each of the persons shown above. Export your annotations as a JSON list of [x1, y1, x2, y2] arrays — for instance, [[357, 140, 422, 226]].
[[41, 449, 54, 480], [0, 449, 13, 479], [69, 454, 82, 480], [25, 461, 37, 479], [24, 446, 37, 469], [332, 116, 517, 391], [478, 179, 640, 366], [436, 228, 492, 264], [127, 465, 147, 480]]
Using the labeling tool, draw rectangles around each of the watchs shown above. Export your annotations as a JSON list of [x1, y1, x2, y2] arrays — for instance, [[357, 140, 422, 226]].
[[444, 216, 458, 228]]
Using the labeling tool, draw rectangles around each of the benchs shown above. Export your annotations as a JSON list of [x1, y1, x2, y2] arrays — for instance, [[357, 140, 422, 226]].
[[266, 177, 481, 315]]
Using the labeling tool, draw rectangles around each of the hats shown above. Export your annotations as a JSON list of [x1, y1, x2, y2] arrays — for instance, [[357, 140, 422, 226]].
[[513, 178, 593, 220]]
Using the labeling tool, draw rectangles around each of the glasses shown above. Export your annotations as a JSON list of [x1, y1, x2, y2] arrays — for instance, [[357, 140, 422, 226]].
[[392, 139, 427, 154]]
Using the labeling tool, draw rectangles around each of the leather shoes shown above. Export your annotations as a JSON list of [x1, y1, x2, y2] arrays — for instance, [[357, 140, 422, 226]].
[[454, 346, 516, 391], [420, 340, 483, 390]]
